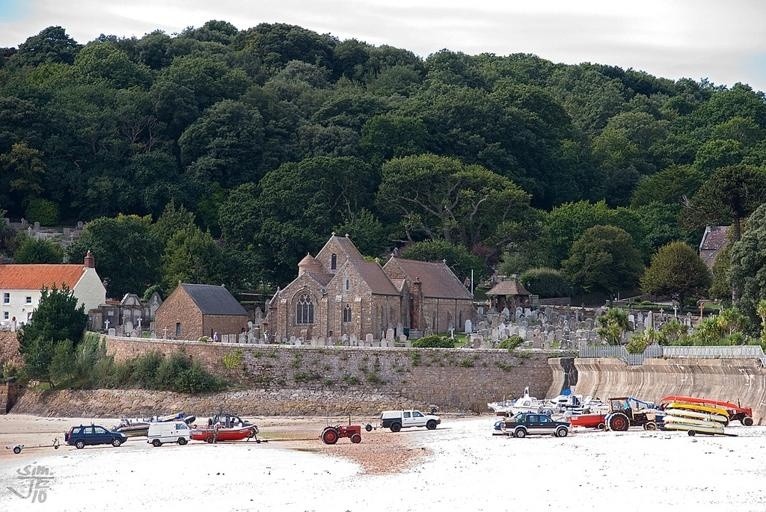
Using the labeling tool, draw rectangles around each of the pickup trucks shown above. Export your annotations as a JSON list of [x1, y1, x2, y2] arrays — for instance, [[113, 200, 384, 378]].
[[208, 413, 249, 427], [379, 408, 441, 432], [501, 414, 572, 438]]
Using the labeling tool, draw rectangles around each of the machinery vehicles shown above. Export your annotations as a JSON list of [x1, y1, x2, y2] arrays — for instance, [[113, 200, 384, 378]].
[[318, 413, 361, 444], [604, 397, 755, 431]]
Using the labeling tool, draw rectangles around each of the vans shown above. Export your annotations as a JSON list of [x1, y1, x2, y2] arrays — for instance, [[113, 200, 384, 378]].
[[147, 421, 192, 447]]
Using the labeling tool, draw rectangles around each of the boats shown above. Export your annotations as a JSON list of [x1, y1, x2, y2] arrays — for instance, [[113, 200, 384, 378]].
[[113, 414, 196, 436], [487, 389, 609, 429], [191, 424, 257, 442]]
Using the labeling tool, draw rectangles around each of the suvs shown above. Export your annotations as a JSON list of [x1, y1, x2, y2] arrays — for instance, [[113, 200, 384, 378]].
[[62, 424, 127, 447]]
